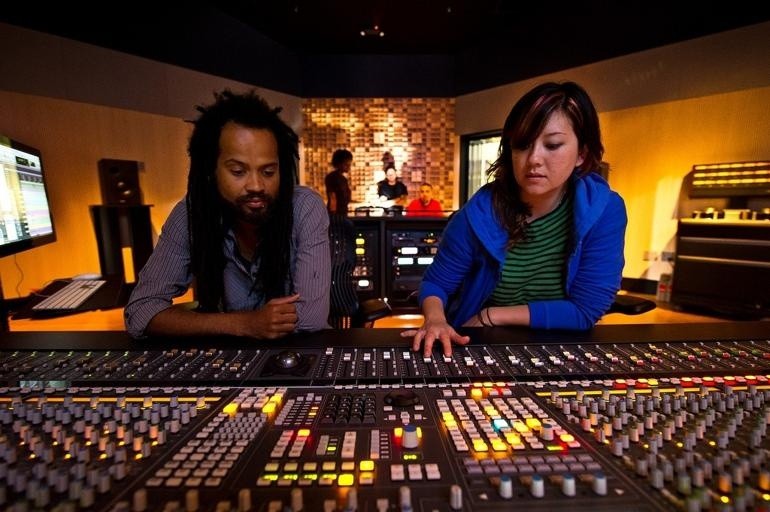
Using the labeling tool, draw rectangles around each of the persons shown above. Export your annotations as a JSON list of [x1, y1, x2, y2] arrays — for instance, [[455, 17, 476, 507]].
[[325, 149, 353, 221], [397, 80, 628, 362], [406, 182, 443, 213], [376, 167, 406, 211], [122, 86, 333, 337]]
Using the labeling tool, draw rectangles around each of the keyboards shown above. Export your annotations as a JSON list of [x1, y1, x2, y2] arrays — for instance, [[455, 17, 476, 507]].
[[32, 280, 106, 310]]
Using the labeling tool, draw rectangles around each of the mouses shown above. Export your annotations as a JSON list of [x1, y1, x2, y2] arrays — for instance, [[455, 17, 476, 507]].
[[72, 274, 102, 280]]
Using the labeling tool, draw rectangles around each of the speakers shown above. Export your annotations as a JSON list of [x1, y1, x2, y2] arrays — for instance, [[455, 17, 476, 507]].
[[97, 159, 141, 207]]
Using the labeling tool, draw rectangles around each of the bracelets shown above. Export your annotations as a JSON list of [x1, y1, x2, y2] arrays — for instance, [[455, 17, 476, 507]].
[[476, 307, 497, 329]]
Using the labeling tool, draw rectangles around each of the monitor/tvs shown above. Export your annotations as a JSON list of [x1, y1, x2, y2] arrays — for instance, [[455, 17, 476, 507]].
[[0, 134, 56, 258]]
